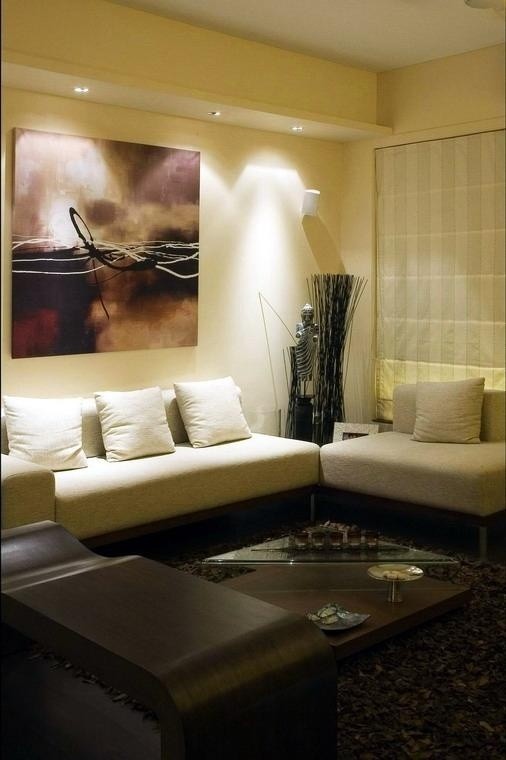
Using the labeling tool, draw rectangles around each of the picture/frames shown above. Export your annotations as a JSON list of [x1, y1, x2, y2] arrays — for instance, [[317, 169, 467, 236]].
[[332, 422, 379, 443], [9, 127, 199, 358]]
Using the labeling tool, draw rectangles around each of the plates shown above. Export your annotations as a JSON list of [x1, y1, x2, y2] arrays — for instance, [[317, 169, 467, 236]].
[[303, 607, 371, 633], [367, 564, 424, 603]]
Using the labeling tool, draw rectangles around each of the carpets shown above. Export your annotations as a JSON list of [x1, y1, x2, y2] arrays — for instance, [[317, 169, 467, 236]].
[[0, 515, 504, 759]]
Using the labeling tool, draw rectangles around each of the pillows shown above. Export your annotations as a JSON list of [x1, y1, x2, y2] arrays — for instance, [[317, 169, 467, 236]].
[[174, 376, 251, 448], [0, 396, 88, 471], [409, 378, 488, 444], [91, 388, 175, 462]]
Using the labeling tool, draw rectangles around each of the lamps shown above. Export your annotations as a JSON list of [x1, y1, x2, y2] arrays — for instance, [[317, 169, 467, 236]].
[[302, 190, 321, 215]]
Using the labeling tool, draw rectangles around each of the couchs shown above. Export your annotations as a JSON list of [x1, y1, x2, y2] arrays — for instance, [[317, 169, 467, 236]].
[[319, 383, 506, 555], [0, 386, 321, 548]]
